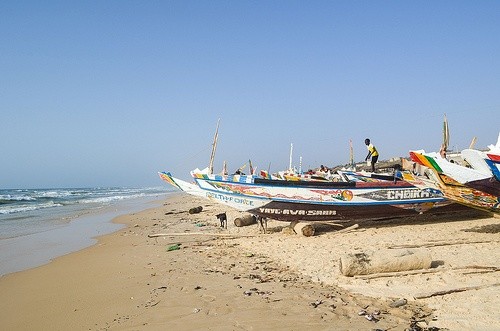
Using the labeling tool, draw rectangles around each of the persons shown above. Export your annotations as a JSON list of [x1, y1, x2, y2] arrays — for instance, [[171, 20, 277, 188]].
[[365, 139, 379, 173], [306, 170, 315, 175], [319, 165, 328, 174]]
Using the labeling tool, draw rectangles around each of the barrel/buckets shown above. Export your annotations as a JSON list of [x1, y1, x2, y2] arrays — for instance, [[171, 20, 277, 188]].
[[294, 222, 315, 236], [234, 214, 256, 226], [189, 205, 202, 214]]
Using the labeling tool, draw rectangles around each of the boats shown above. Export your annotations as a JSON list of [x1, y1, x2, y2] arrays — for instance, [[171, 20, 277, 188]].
[[158, 117, 499, 221]]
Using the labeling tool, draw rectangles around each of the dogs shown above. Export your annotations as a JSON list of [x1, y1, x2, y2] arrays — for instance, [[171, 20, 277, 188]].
[[215, 211, 228, 229]]
